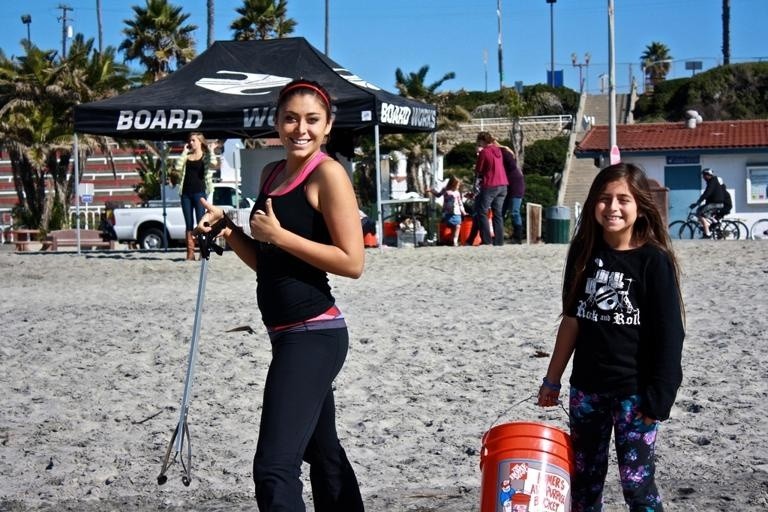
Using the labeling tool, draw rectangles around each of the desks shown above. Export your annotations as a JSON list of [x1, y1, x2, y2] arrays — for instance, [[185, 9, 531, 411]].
[[381, 198, 431, 249], [13, 228, 41, 251]]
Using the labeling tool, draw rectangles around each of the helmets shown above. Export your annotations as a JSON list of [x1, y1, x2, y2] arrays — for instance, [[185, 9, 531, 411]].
[[701, 169, 715, 176]]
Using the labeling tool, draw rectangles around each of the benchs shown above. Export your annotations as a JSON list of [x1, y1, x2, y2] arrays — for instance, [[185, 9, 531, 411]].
[[48, 229, 110, 251]]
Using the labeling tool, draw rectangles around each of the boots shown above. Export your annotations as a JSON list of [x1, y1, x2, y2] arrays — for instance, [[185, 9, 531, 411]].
[[186, 230, 195, 260], [506, 223, 523, 244]]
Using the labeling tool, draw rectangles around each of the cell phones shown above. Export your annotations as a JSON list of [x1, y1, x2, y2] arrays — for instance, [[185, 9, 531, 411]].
[[186, 143, 191, 149]]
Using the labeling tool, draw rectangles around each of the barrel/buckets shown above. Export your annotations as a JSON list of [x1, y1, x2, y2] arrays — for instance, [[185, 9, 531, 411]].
[[547, 206, 570, 243], [480, 393, 574, 512]]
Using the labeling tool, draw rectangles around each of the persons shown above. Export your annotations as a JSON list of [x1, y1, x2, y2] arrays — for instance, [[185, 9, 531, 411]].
[[174, 133, 226, 261], [423, 172, 471, 248], [191, 77, 370, 511], [471, 129, 511, 247], [707, 175, 733, 231], [487, 137, 527, 244], [534, 159, 688, 512], [687, 167, 727, 240], [458, 142, 493, 248]]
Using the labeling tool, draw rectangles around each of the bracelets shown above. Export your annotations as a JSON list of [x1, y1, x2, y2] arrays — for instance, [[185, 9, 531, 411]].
[[542, 376, 562, 392]]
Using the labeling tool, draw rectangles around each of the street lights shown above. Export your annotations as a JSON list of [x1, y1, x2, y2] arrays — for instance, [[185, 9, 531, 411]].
[[21, 14, 32, 48], [546, 0, 557, 88], [572, 52, 591, 91]]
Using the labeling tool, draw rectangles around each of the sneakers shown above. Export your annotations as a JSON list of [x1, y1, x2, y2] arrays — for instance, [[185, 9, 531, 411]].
[[700, 233, 712, 239]]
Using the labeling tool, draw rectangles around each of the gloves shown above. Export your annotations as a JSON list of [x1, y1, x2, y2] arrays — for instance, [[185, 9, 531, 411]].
[[690, 204, 697, 209]]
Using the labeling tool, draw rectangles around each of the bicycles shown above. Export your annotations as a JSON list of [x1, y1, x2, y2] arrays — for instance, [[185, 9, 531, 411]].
[[668, 203, 768, 241]]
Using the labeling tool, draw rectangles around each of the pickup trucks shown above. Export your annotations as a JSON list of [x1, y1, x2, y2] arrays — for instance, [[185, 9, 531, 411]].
[[111, 183, 256, 251]]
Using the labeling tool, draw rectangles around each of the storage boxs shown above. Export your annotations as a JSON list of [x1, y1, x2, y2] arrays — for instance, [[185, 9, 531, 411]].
[[394, 230, 427, 248]]
